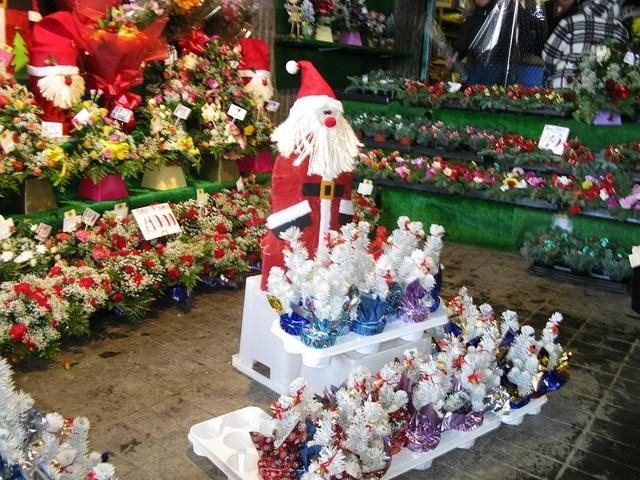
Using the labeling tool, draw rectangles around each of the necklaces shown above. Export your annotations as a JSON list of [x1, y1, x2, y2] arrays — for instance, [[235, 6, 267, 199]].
[[552, 1, 570, 17]]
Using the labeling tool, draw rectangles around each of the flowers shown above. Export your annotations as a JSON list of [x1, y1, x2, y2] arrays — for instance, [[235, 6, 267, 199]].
[[0, 0, 640, 480]]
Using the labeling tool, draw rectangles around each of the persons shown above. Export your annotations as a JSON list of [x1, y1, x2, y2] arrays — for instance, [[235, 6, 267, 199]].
[[233, 36, 273, 102], [540, 0, 630, 89], [452, 0, 543, 97], [547, 0, 581, 44], [24, 11, 99, 138], [261, 57, 370, 300]]
